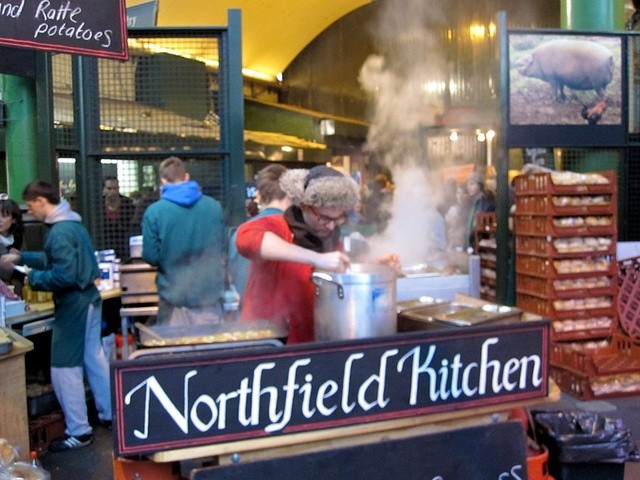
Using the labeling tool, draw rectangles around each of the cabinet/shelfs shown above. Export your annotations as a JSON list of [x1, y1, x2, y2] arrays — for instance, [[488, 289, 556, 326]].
[[512, 169, 640, 410], [474, 211, 496, 301]]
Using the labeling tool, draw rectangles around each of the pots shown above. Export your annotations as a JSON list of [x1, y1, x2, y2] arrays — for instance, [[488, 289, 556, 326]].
[[312, 262, 397, 340]]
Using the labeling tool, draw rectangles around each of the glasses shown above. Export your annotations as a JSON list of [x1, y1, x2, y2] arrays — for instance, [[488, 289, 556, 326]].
[[308, 204, 347, 225], [0, 193, 8, 200]]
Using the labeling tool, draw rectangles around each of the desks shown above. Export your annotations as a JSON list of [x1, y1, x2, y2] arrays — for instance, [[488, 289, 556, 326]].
[[120, 306, 160, 359]]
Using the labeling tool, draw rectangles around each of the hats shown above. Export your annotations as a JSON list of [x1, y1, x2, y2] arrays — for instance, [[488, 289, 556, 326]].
[[279, 165, 360, 208]]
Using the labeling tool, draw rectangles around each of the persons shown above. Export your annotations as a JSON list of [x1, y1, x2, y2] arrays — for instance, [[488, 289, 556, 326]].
[[0, 180, 112, 453], [435, 170, 494, 253], [0, 199, 25, 300], [235, 166, 362, 345], [96, 176, 136, 264], [227, 163, 294, 315], [142, 158, 230, 325]]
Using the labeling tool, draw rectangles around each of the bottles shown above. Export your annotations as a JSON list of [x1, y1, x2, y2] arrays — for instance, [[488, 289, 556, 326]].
[[101, 263, 113, 289], [27, 453, 42, 469]]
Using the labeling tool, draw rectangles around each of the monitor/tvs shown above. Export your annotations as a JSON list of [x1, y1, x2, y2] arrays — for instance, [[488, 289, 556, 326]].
[[507, 27, 640, 146]]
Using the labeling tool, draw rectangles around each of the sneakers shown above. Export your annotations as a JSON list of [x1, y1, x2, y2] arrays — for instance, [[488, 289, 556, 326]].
[[47, 434, 92, 451]]
